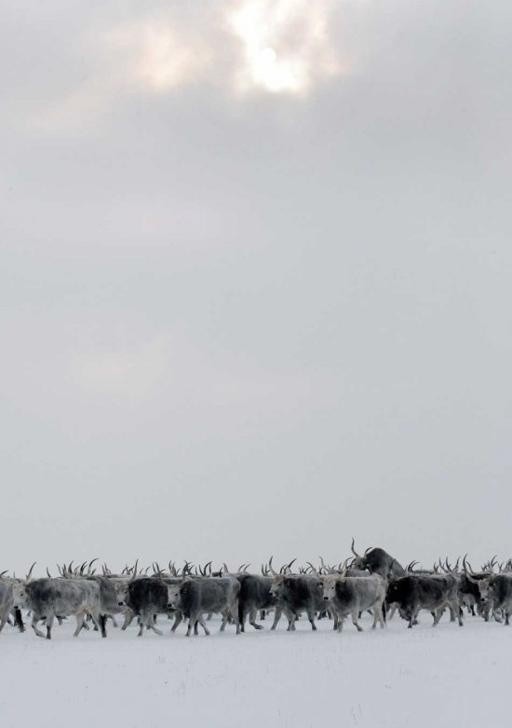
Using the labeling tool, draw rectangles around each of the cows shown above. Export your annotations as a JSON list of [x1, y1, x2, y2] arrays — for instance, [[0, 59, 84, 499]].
[[352, 535, 404, 583], [0, 553, 512, 639]]
